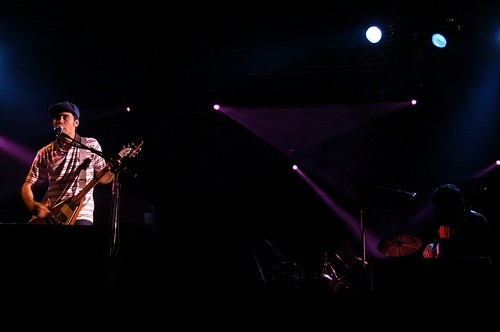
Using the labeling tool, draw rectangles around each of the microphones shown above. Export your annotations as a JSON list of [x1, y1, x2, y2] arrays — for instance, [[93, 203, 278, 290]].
[[401, 191, 417, 199], [50, 126, 64, 139]]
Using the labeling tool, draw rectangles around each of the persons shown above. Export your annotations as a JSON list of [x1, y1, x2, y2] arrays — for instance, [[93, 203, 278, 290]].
[[423, 184, 491, 259], [21, 101, 142, 225]]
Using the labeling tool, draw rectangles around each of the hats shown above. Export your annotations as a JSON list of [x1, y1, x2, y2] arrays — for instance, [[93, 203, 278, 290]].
[[48, 102, 80, 119]]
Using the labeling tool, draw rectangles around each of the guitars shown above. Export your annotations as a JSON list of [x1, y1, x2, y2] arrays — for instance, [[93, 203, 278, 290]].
[[29, 140, 144, 224]]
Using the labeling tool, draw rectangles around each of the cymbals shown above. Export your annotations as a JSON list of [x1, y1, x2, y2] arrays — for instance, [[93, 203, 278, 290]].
[[377, 233, 422, 257]]
